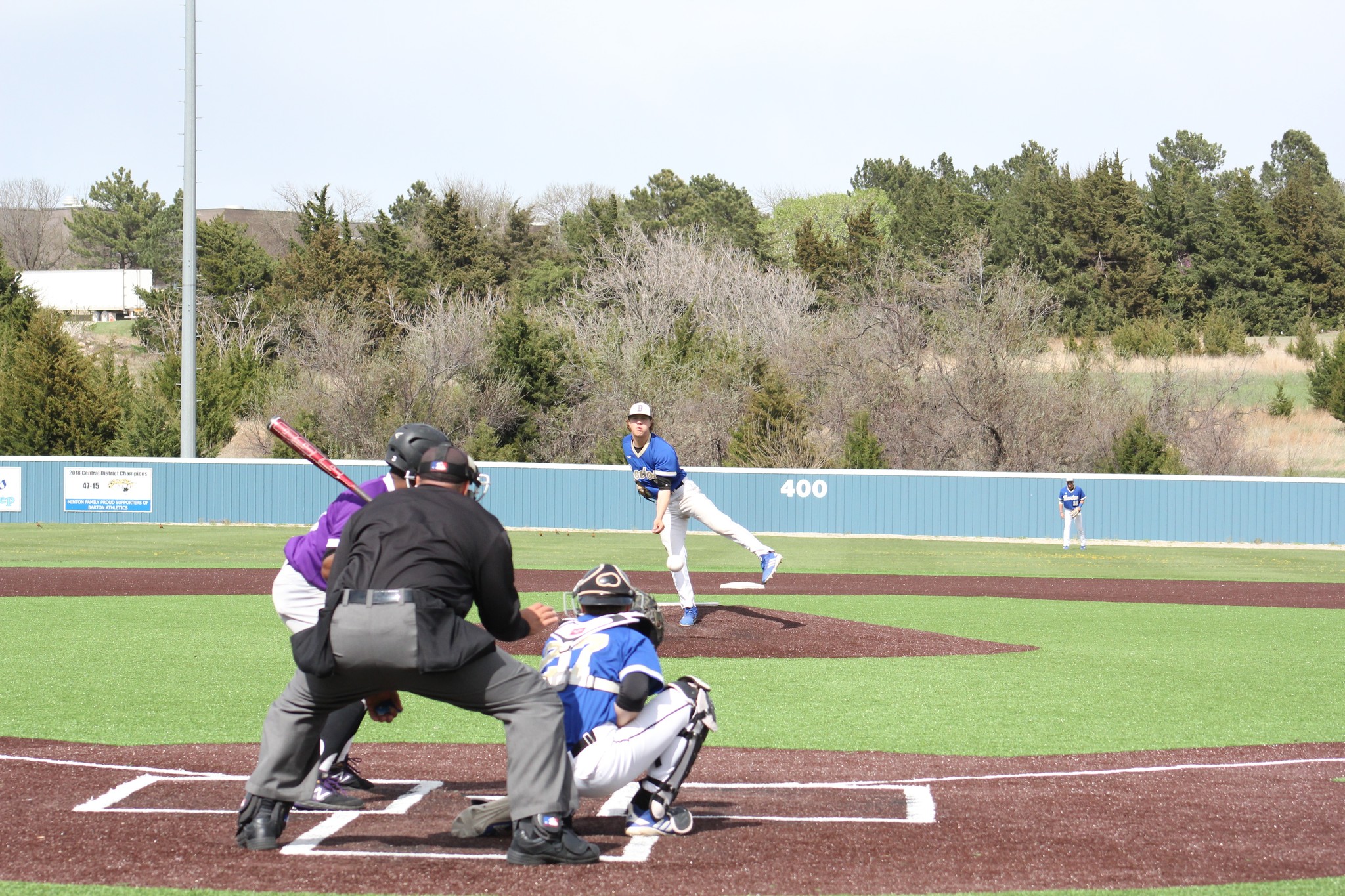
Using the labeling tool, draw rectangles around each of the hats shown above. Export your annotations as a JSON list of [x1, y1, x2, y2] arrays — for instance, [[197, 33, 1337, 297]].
[[416, 442, 481, 487], [627, 402, 653, 418], [1066, 476, 1073, 482]]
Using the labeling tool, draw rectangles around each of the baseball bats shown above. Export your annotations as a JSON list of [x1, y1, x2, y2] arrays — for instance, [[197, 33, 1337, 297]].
[[266, 414, 371, 503]]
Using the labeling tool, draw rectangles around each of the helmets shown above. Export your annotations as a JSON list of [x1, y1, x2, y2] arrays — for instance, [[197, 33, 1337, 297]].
[[384, 423, 452, 478]]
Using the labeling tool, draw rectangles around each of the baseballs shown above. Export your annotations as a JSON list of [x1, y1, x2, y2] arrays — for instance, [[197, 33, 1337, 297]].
[[667, 554, 684, 572]]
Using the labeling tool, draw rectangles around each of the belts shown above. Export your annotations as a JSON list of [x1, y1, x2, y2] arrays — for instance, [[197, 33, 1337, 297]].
[[670, 481, 684, 495], [340, 588, 415, 604], [568, 731, 596, 757]]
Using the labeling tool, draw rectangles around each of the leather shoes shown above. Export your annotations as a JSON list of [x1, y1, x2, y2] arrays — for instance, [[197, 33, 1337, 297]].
[[235, 816, 278, 851], [507, 828, 600, 865]]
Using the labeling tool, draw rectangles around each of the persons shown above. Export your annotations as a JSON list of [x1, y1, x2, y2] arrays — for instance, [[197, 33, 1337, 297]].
[[621, 401, 783, 628], [451, 562, 718, 838], [1058, 474, 1086, 550], [234, 446, 601, 864], [269, 424, 452, 810]]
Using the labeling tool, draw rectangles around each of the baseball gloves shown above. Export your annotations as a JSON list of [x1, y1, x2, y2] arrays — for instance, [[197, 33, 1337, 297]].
[[1069, 508, 1081, 518], [626, 589, 664, 648], [634, 479, 655, 500]]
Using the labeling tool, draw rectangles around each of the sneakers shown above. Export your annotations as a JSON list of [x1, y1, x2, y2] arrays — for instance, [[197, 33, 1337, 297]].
[[1080, 546, 1086, 550], [1063, 546, 1068, 549], [624, 801, 692, 835], [293, 777, 364, 809], [452, 798, 510, 839], [322, 755, 375, 790], [758, 551, 783, 584], [679, 606, 698, 627]]
